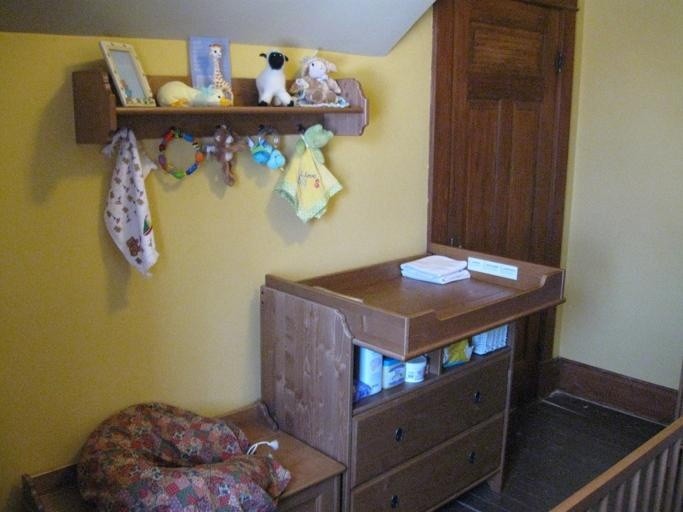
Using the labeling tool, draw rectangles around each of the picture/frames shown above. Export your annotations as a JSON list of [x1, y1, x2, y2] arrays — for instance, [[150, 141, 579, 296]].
[[98, 41, 157, 108]]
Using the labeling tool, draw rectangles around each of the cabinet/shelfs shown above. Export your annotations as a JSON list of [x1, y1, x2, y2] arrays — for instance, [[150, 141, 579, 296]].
[[260, 244, 569, 512], [22, 400, 347, 511]]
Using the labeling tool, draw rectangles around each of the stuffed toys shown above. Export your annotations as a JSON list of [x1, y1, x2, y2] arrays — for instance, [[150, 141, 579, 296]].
[[290, 58, 350, 108], [255, 49, 294, 107], [155, 81, 232, 108]]
